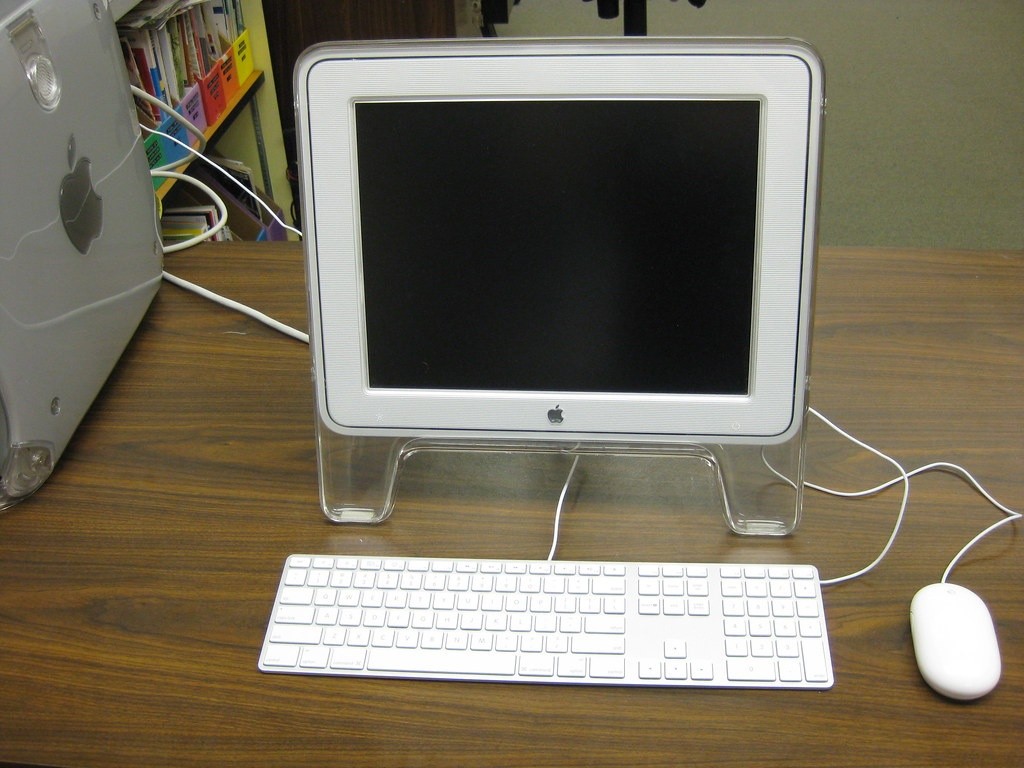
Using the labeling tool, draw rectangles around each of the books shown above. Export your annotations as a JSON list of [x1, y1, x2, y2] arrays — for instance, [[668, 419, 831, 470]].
[[115, 0, 245, 140], [161, 155, 263, 243]]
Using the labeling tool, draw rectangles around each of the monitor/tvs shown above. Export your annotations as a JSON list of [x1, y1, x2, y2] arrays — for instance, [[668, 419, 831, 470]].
[[293, 35, 828, 536]]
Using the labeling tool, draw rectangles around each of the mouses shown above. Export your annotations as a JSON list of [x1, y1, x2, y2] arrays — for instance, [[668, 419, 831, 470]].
[[909, 582, 1001, 700]]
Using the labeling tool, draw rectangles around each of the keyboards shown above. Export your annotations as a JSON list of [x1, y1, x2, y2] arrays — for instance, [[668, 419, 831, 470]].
[[258, 554, 835, 691]]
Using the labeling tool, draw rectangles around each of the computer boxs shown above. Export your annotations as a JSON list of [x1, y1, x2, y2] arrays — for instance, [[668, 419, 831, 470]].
[[0, 0, 165, 507]]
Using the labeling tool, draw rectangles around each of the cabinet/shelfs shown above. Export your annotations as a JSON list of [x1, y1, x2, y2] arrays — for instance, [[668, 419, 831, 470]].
[[110, 0, 296, 241]]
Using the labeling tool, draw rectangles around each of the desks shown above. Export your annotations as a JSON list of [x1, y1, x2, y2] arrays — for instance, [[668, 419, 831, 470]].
[[0, 246, 1024, 768]]
